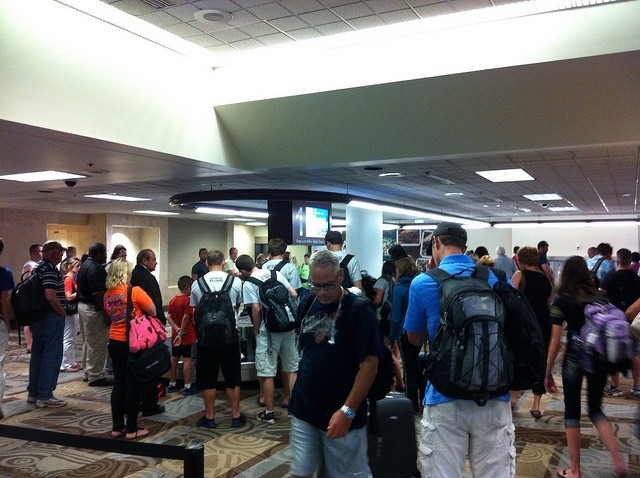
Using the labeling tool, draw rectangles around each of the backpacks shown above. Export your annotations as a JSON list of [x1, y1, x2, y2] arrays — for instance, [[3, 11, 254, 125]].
[[572, 300, 633, 377], [12, 273, 48, 326], [424, 277, 516, 406], [258, 278, 299, 332], [349, 295, 395, 399], [194, 290, 238, 348], [494, 267, 547, 389]]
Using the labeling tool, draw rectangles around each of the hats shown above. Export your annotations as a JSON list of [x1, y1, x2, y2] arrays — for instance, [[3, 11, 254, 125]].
[[426, 222, 467, 256], [41, 240, 67, 251]]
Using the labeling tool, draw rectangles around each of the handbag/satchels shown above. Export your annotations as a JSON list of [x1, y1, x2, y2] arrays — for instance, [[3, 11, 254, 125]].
[[129, 311, 166, 353], [123, 341, 171, 385]]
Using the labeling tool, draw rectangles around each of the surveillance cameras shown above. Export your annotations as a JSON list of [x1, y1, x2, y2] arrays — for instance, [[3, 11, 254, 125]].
[[63, 179, 77, 187]]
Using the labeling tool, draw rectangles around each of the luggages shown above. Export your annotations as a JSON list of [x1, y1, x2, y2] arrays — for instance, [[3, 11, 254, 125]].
[[368, 392, 421, 477]]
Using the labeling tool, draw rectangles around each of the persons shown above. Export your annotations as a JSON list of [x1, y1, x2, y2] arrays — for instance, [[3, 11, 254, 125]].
[[402, 221, 517, 477], [77, 242, 115, 387], [324, 231, 363, 289], [189, 250, 246, 428], [166, 275, 197, 396], [21, 242, 43, 282], [586, 243, 616, 289], [388, 243, 409, 260], [372, 259, 405, 392], [471, 245, 493, 266], [386, 257, 427, 415], [26, 241, 69, 408], [297, 253, 313, 291], [223, 247, 242, 277], [191, 248, 209, 280], [287, 249, 385, 478], [544, 255, 629, 478], [493, 245, 519, 280], [60, 245, 77, 268], [60, 257, 83, 373], [538, 242, 556, 286], [0, 238, 16, 420], [102, 259, 157, 440], [510, 245, 552, 418], [131, 249, 167, 416], [630, 252, 640, 274], [598, 248, 640, 398], [512, 245, 520, 269], [236, 254, 299, 425], [261, 237, 303, 295], [110, 245, 127, 260]]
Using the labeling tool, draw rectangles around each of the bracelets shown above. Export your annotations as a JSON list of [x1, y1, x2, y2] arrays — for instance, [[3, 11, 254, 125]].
[[178, 330, 186, 338]]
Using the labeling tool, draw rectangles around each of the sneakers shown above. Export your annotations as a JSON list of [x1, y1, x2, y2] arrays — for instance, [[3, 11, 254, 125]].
[[182, 382, 200, 394], [198, 416, 217, 428], [167, 381, 181, 393], [256, 409, 275, 424], [36, 397, 67, 408]]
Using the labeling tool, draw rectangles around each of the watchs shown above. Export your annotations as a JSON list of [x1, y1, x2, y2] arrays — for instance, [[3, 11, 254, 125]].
[[341, 405, 356, 420]]
